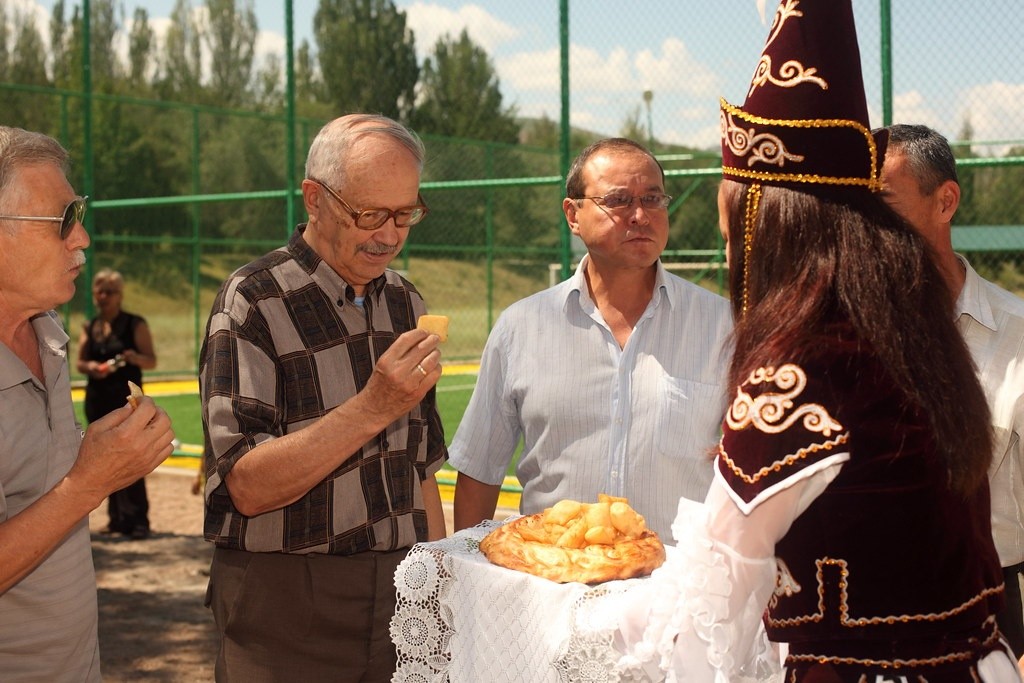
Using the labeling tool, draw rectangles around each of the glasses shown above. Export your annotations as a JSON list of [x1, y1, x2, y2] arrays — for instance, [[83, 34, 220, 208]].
[[570, 191, 672, 209], [308, 178, 429, 230], [93, 288, 121, 297], [0, 193, 88, 241]]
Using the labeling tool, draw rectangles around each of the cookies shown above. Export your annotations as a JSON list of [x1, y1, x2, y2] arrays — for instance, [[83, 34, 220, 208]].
[[126, 380, 143, 410]]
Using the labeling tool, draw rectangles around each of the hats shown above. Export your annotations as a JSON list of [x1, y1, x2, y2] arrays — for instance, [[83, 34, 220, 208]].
[[719, 0, 892, 193]]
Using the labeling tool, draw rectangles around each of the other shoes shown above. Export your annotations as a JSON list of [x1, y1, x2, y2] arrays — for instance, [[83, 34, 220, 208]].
[[90, 524, 148, 542]]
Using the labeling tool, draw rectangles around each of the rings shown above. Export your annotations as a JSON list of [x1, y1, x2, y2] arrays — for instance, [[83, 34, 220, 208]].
[[417, 363, 427, 376]]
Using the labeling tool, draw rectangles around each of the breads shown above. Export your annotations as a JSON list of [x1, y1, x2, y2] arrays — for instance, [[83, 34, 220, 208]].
[[478, 493, 666, 585], [416, 315, 448, 342]]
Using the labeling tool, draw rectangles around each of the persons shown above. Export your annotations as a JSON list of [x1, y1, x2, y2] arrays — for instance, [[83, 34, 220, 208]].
[[0, 123, 177, 683], [610, 0, 1023, 683], [198, 112, 451, 683], [77, 268, 158, 540], [444, 137, 738, 546]]
